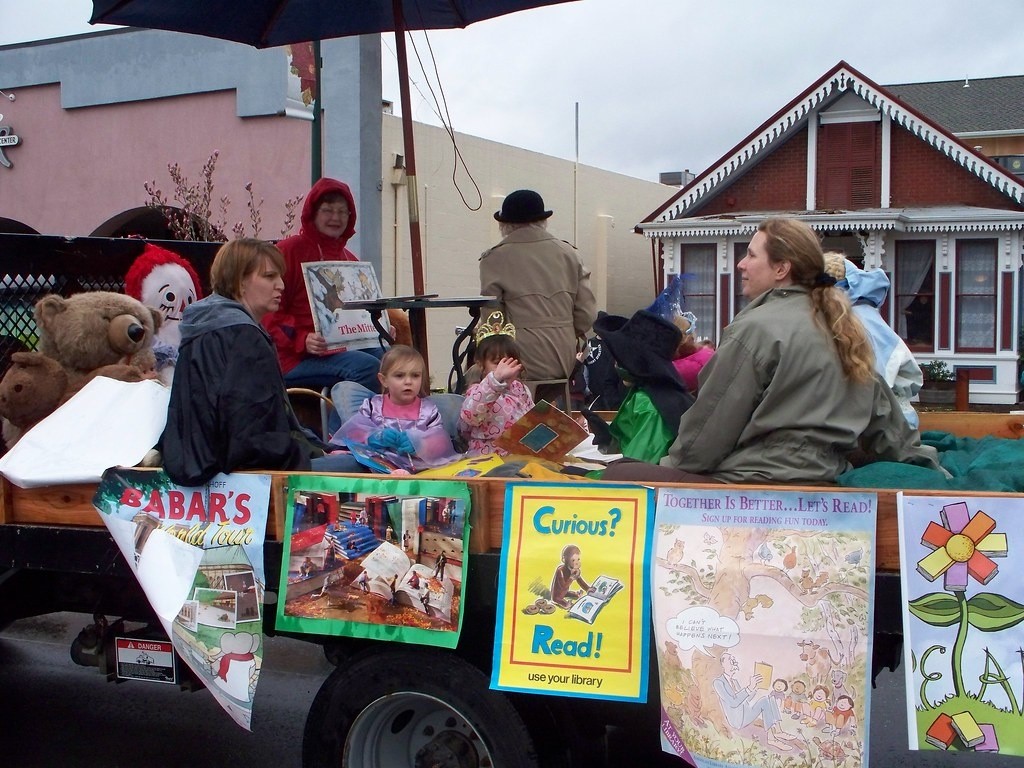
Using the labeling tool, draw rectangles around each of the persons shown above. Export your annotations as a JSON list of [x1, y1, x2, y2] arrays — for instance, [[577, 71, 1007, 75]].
[[823, 251, 923, 432], [480, 189, 597, 404], [330, 346, 464, 473], [458, 310, 536, 460], [260, 176, 396, 443], [602, 215, 953, 485], [560, 275, 716, 480], [163, 237, 365, 473]]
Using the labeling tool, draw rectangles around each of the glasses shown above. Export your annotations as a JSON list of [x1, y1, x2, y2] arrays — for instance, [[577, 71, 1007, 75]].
[[317, 207, 351, 216]]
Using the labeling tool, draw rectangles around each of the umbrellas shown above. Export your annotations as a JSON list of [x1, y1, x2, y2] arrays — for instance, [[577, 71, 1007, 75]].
[[88, 0, 585, 395]]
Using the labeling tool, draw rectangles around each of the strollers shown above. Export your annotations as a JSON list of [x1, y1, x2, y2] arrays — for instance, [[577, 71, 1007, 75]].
[[582, 309, 697, 456]]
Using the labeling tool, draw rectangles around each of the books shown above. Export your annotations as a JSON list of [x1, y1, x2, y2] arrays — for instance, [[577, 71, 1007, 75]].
[[294, 491, 454, 625]]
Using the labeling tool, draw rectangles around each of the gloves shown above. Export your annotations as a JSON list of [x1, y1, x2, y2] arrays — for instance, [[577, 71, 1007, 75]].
[[581, 408, 611, 445], [395, 430, 415, 455], [369, 428, 400, 448], [560, 465, 592, 477]]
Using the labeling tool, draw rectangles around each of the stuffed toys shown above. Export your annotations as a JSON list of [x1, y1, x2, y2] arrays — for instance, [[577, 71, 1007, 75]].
[[0, 243, 204, 471]]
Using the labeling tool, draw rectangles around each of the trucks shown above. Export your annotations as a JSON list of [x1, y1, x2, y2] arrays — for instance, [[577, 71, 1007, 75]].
[[0, 234, 1024, 768]]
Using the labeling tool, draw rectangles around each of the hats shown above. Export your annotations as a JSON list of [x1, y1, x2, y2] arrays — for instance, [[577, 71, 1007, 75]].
[[592, 310, 688, 391], [494, 189, 553, 224]]
